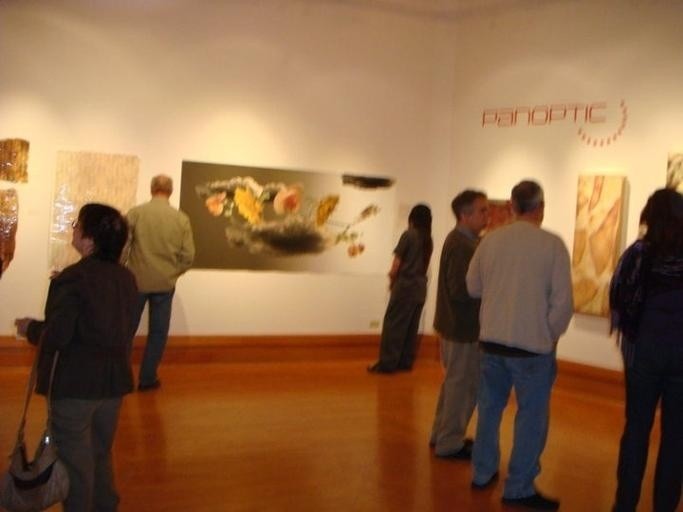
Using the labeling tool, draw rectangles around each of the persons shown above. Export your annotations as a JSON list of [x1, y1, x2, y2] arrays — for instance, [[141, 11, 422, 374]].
[[463, 178, 574, 511], [426, 186, 493, 465], [12, 203, 138, 511], [607, 187, 682, 511], [122, 173, 196, 397], [365, 201, 433, 374]]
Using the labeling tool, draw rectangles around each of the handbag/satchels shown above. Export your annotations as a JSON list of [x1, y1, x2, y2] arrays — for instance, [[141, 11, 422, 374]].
[[1, 429, 72, 511]]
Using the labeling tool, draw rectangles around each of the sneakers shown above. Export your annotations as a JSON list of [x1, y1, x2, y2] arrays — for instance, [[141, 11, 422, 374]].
[[472, 472, 498, 491], [135, 380, 161, 392], [367, 362, 415, 374], [428, 437, 474, 459], [502, 491, 560, 511]]
